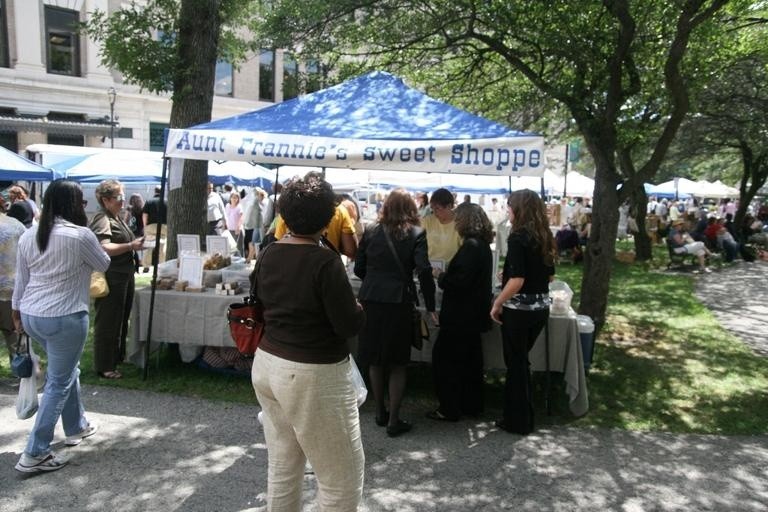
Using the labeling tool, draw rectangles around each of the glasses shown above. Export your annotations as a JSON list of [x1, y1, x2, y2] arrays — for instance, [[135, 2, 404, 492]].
[[82, 199, 86, 206], [430, 206, 447, 211], [110, 194, 124, 202]]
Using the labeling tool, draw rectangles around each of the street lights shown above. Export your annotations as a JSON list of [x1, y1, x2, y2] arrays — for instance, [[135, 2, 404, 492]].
[[108, 86, 116, 148]]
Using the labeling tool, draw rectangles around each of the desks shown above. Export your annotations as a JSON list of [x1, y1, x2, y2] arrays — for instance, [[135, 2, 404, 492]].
[[126, 281, 590, 419]]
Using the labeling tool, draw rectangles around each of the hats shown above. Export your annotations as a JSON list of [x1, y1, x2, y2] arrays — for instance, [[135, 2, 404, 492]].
[[672, 220, 684, 226], [16, 180, 29, 194]]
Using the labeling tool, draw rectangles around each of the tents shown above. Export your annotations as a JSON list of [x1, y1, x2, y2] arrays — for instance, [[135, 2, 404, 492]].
[[142, 69, 553, 417]]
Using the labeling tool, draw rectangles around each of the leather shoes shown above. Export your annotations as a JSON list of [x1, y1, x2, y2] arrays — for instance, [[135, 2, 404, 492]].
[[143, 267, 147, 272]]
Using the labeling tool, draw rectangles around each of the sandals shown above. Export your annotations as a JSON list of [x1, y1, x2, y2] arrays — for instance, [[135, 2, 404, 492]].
[[427, 411, 445, 419], [99, 370, 122, 379], [387, 420, 413, 437], [376, 411, 390, 424]]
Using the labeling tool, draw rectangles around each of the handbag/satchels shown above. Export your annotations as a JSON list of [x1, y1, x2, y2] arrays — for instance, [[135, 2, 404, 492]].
[[382, 222, 429, 351], [11, 332, 34, 378], [88, 209, 109, 297], [126, 206, 137, 232], [227, 241, 273, 355]]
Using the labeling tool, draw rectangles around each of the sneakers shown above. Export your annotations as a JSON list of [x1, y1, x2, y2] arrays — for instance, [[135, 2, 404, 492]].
[[64, 424, 97, 446], [699, 268, 711, 273], [14, 454, 68, 472], [712, 253, 720, 259]]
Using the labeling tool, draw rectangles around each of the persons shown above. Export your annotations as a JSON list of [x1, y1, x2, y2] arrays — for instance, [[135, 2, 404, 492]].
[[575, 197, 585, 232], [353, 186, 439, 437], [7, 185, 40, 229], [559, 197, 572, 230], [243, 186, 269, 264], [490, 188, 556, 436], [668, 220, 722, 274], [207, 182, 228, 237], [646, 191, 739, 223], [225, 192, 246, 258], [219, 181, 234, 206], [420, 188, 465, 264], [0, 191, 27, 382], [123, 193, 144, 265], [724, 213, 757, 262], [16, 184, 40, 226], [426, 202, 495, 421], [703, 217, 744, 263], [263, 181, 283, 233], [88, 178, 146, 380], [273, 170, 358, 258], [142, 185, 168, 241], [248, 176, 365, 512], [585, 198, 591, 208], [9, 179, 112, 474]]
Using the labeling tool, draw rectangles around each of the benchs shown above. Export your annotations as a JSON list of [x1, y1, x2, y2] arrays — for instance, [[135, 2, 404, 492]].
[[703, 235, 735, 268], [666, 239, 706, 270]]
